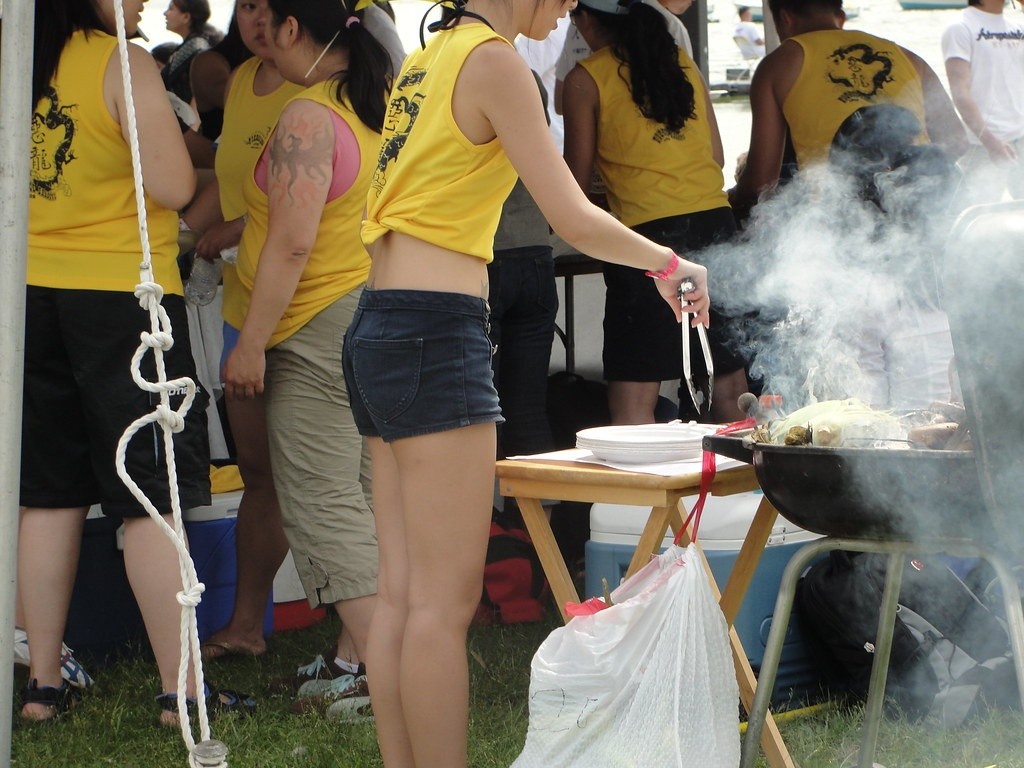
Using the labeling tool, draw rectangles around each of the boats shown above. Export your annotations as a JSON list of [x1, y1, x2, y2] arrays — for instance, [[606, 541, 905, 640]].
[[731, 2, 861, 23], [710, 39, 766, 94], [707, 3, 720, 23], [897, 0, 1012, 10]]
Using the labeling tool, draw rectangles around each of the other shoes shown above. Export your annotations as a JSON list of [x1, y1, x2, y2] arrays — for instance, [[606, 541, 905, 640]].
[[294, 654, 379, 726], [13, 624, 99, 694]]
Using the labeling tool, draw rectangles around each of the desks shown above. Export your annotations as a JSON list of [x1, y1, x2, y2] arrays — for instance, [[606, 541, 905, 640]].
[[493, 421, 796, 768]]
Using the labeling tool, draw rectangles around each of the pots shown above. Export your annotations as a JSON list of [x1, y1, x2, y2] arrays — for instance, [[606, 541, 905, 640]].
[[703, 431, 990, 536]]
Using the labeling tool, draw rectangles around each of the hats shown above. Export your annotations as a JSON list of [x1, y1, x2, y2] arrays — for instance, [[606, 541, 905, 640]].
[[579, 0, 632, 15]]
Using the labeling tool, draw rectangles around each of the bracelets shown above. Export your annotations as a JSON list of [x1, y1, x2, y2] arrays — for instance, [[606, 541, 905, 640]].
[[645, 252, 679, 281]]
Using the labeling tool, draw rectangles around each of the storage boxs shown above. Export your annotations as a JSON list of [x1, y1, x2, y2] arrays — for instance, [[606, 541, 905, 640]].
[[584, 489, 829, 714], [181, 486, 327, 646]]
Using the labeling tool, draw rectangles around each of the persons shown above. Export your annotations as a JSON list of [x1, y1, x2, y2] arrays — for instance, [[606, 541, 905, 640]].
[[733, 6, 766, 59], [344, 0, 710, 768], [727, 0, 970, 208], [486, 0, 749, 580], [18, 0, 407, 731], [942, 0, 1024, 202]]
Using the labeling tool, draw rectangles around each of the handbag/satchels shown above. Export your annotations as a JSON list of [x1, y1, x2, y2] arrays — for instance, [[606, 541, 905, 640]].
[[473, 506, 558, 622], [508, 542, 741, 768]]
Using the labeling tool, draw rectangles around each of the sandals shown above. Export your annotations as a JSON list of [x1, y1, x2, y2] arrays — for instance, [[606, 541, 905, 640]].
[[13, 679, 98, 731], [155, 680, 259, 731]]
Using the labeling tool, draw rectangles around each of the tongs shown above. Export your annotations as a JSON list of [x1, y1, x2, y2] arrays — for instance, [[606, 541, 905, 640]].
[[676, 277, 715, 416]]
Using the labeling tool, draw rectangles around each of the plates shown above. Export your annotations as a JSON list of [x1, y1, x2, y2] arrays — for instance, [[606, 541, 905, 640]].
[[576, 424, 726, 464]]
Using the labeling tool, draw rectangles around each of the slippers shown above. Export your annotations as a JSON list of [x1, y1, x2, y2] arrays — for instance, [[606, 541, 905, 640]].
[[200, 632, 269, 669]]
